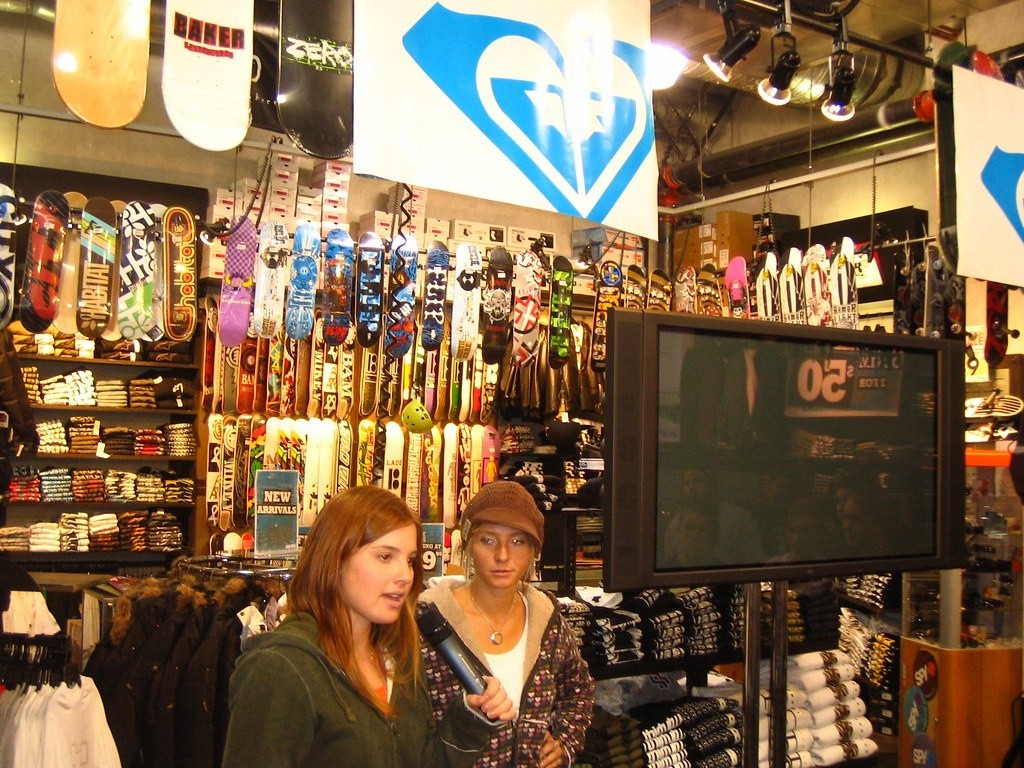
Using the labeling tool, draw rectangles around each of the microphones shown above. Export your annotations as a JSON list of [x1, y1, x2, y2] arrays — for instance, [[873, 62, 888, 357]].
[[419, 607, 500, 724]]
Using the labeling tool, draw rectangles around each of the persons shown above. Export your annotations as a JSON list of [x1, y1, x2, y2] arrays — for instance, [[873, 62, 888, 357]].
[[223, 484, 515, 768], [658, 444, 938, 566], [414, 482, 597, 768]]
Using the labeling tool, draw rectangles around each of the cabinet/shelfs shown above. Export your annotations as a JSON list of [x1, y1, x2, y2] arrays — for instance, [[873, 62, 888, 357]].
[[501, 452, 602, 518], [896, 448, 1024, 768], [549, 504, 765, 768], [761, 579, 898, 768], [0, 319, 208, 567]]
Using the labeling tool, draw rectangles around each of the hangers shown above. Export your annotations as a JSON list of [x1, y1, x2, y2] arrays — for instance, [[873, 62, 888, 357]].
[[0, 632, 82, 695], [175, 552, 284, 613]]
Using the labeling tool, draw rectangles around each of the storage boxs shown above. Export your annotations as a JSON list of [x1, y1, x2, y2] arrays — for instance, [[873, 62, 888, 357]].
[[672, 210, 800, 271], [356, 183, 647, 297], [200, 148, 353, 290]]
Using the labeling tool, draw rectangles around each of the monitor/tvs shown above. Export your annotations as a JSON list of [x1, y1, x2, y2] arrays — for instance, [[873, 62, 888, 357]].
[[604, 307, 970, 594]]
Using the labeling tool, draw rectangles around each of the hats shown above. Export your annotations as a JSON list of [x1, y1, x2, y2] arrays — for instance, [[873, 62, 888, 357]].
[[461, 481, 544, 561]]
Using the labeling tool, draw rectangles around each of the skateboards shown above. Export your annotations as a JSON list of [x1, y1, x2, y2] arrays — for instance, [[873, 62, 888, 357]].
[[100, 200, 123, 342], [52, 192, 88, 334], [890, 38, 1024, 445], [117, 201, 155, 340], [0, 183, 18, 329], [161, 0, 354, 160], [51, 0, 151, 130], [161, 205, 197, 342], [19, 190, 70, 333], [140, 203, 167, 343], [203, 213, 860, 576], [76, 196, 117, 340]]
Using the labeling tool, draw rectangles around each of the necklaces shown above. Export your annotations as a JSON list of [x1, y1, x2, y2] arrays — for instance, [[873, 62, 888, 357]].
[[368, 647, 375, 667], [466, 584, 519, 644]]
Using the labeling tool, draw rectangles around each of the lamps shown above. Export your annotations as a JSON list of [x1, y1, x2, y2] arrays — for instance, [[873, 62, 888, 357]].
[[757, 47, 801, 106], [820, 64, 857, 122], [704, 26, 758, 82]]
